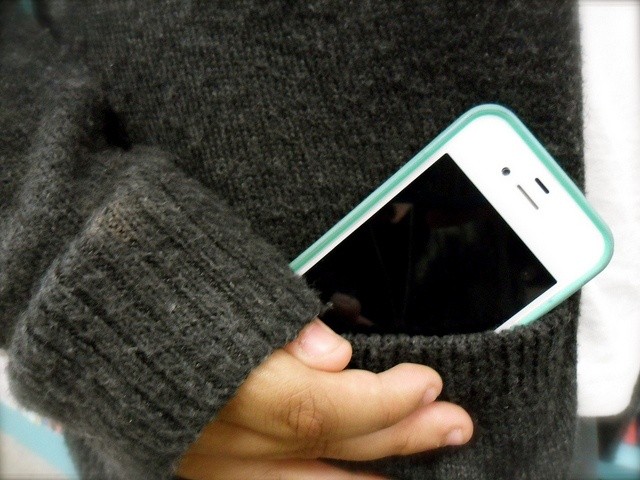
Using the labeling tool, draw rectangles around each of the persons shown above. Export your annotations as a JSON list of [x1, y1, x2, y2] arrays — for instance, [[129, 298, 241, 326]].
[[0, 2, 585, 478]]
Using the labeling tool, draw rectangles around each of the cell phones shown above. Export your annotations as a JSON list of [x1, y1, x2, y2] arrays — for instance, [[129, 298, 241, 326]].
[[285, 103, 614, 334]]
